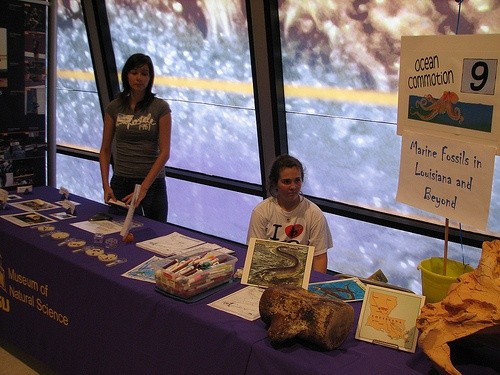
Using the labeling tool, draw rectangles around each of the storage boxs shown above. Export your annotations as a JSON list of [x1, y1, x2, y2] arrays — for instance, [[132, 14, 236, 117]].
[[150, 247, 239, 301]]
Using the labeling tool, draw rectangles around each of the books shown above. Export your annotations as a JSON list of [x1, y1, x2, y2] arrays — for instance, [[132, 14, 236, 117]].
[[0, 194, 426, 353], [0, 0, 46, 187]]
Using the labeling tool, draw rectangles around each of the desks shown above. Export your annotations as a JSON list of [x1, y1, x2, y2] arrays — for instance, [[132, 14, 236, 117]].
[[0, 185, 441, 375]]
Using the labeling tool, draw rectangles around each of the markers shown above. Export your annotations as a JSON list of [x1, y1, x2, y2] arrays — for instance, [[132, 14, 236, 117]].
[[155, 251, 233, 298]]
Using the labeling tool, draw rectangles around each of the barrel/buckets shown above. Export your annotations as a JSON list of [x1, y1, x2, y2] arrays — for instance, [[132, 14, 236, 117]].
[[416, 257, 474, 304]]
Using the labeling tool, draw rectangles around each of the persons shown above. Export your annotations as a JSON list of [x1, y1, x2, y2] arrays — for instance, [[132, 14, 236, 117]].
[[246, 155, 333, 274], [99, 54, 171, 223]]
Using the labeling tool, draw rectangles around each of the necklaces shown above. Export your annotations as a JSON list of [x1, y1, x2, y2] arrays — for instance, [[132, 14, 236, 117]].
[[276, 196, 300, 223]]
